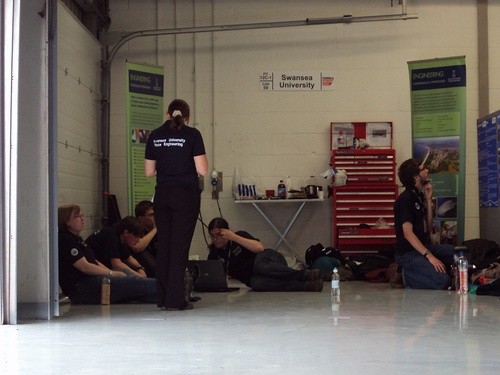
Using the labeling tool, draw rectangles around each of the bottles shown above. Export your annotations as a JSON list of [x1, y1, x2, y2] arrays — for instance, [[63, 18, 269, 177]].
[[456, 252, 469, 294], [101, 277, 111, 305], [330, 268, 340, 296], [278, 180, 286, 200]]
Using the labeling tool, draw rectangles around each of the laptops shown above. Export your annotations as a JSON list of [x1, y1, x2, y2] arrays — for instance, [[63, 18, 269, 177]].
[[187, 259, 241, 293]]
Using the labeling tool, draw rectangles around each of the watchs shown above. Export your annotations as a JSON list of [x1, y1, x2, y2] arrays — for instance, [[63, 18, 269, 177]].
[[137, 267, 145, 270], [109, 270, 113, 276]]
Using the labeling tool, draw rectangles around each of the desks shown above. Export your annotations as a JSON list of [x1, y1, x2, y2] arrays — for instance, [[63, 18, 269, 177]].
[[233, 198, 325, 257]]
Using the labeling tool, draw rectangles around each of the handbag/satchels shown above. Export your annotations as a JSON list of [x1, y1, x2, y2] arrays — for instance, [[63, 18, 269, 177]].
[[304, 242, 396, 282], [449, 236, 500, 296]]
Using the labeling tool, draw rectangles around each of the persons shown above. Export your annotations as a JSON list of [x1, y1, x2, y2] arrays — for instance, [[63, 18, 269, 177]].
[[386, 158, 452, 289], [206, 217, 324, 293], [58, 99, 209, 311]]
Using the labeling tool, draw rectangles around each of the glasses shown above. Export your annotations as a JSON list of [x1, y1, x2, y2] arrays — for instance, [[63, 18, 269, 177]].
[[73, 214, 84, 219]]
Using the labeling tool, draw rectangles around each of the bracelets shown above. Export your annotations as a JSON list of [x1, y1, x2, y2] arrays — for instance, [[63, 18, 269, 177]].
[[424, 249, 430, 257]]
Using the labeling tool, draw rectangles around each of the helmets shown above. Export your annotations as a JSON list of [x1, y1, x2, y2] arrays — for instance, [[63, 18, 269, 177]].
[[397, 157, 422, 188]]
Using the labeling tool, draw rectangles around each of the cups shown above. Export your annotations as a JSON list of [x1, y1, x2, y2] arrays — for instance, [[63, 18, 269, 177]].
[[304, 184, 318, 199]]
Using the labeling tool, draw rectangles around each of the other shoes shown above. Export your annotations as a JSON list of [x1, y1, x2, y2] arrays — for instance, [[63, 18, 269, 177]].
[[387, 262, 404, 288], [304, 268, 324, 292]]
